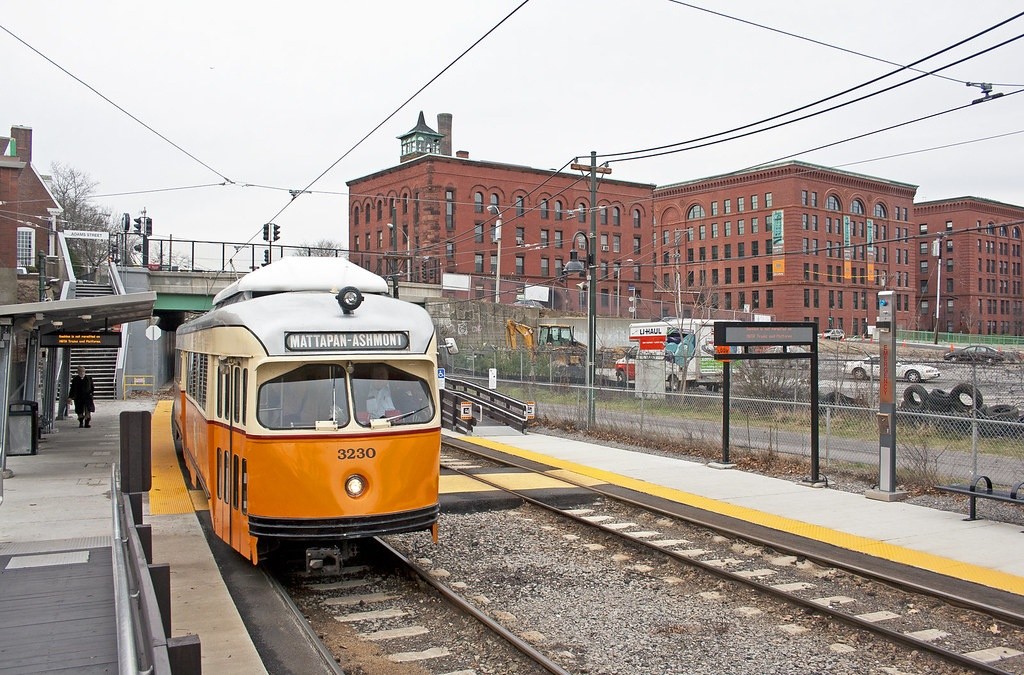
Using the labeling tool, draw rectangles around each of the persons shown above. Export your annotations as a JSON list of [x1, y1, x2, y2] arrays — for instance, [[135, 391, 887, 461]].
[[68, 367, 95, 427], [359, 362, 413, 419]]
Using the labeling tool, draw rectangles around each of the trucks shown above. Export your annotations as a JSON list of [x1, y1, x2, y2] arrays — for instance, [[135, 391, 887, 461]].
[[615, 316, 748, 389]]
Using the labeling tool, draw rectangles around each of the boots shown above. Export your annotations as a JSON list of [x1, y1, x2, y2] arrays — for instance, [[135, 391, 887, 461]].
[[77, 415, 84, 428], [85, 418, 92, 428]]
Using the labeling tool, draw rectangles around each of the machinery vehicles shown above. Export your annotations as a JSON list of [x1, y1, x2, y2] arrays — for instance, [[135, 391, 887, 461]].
[[501, 316, 588, 381]]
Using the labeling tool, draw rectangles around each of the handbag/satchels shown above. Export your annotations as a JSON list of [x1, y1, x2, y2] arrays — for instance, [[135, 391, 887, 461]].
[[86, 392, 96, 412]]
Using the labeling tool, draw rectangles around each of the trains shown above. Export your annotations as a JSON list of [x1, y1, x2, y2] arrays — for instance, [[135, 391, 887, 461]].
[[172, 249, 458, 575]]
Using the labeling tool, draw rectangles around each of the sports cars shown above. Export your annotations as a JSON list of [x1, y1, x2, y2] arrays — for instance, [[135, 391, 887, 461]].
[[842, 354, 941, 383]]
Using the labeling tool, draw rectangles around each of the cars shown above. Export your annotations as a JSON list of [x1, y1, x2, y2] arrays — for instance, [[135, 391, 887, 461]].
[[943, 344, 1005, 366], [749, 343, 811, 371], [817, 328, 842, 340]]
